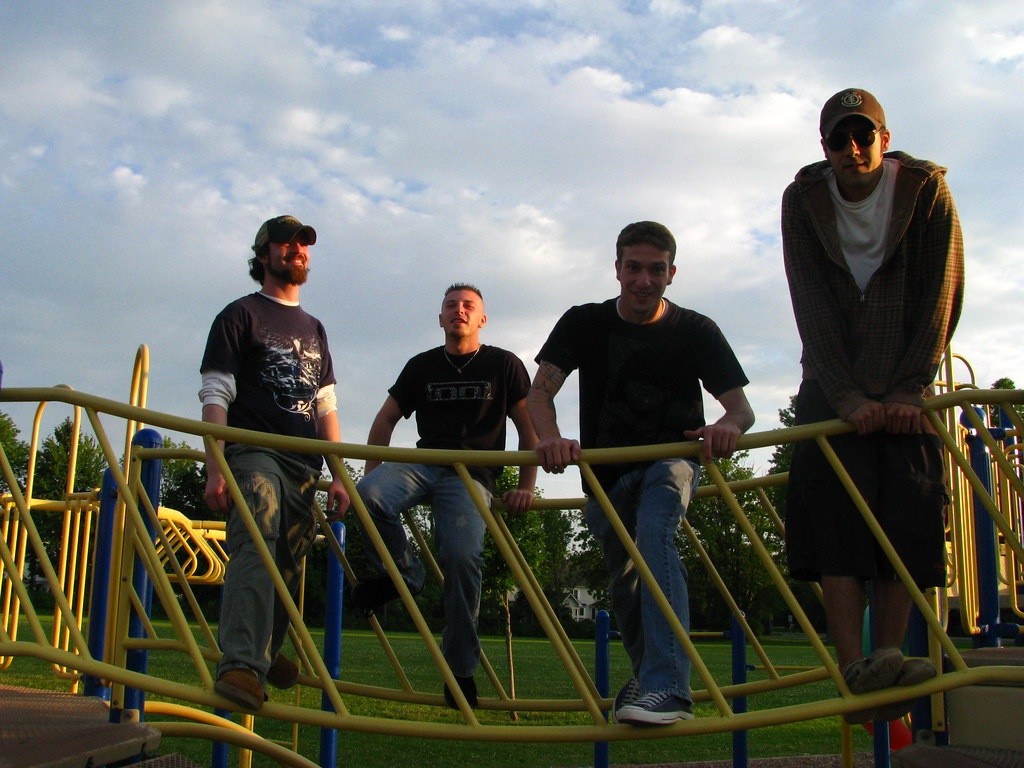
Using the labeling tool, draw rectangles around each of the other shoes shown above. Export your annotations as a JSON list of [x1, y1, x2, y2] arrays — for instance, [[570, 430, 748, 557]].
[[443, 672, 479, 711], [353, 573, 401, 609], [268, 651, 300, 688], [841, 647, 903, 725], [881, 659, 936, 721], [216, 668, 265, 710]]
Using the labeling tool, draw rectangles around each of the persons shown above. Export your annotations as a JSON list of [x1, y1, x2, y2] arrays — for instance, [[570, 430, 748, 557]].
[[780, 89, 968, 724], [199, 214, 348, 708], [357, 285, 541, 706], [525, 221, 755, 729]]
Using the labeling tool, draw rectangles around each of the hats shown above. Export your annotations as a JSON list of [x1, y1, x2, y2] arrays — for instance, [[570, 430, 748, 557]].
[[820, 88, 884, 137], [255, 216, 316, 252]]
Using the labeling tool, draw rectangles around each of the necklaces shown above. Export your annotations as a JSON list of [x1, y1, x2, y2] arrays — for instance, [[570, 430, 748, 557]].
[[444, 342, 481, 374]]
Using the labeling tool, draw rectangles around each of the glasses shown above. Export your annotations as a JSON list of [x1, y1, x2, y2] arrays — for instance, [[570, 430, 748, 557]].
[[824, 129, 875, 153]]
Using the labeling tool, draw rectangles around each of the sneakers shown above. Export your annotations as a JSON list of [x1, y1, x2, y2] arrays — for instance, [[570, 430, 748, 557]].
[[618, 691, 694, 723], [612, 678, 644, 722]]
